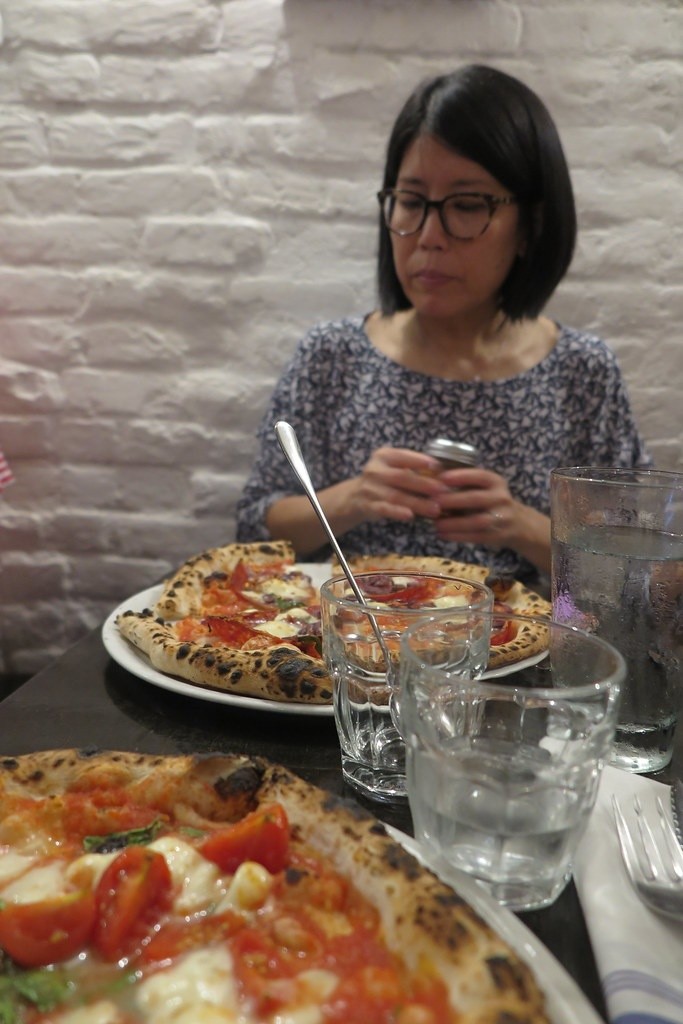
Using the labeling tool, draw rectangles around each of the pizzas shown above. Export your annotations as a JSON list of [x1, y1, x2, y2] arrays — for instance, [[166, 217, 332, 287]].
[[114, 541, 553, 706], [0, 747, 553, 1024]]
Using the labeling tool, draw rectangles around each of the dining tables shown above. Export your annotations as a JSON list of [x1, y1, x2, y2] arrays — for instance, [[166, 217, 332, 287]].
[[1, 598, 683, 1024]]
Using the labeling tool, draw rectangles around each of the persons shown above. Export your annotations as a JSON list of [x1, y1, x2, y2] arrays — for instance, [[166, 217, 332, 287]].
[[229, 64, 658, 602]]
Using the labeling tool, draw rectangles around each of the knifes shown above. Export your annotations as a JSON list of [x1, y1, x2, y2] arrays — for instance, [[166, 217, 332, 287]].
[[670, 777, 683, 848]]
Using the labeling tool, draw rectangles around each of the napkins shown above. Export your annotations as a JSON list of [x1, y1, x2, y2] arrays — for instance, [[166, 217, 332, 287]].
[[575, 762, 682, 1024]]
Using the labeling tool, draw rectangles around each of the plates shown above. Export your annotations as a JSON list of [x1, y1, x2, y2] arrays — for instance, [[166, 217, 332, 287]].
[[358, 810, 614, 1024], [102, 556, 566, 715]]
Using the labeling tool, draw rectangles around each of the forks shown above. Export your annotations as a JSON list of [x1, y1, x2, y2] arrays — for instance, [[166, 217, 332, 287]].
[[610, 787, 683, 926]]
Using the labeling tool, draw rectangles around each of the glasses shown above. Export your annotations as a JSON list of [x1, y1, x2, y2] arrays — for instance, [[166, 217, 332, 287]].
[[378, 188, 517, 241]]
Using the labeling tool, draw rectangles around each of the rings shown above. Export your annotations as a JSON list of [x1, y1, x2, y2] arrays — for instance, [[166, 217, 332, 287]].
[[490, 511, 504, 523]]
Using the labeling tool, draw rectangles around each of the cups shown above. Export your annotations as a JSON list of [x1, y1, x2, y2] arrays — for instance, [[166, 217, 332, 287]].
[[546, 463, 683, 774], [391, 608, 625, 915], [314, 568, 493, 807]]
